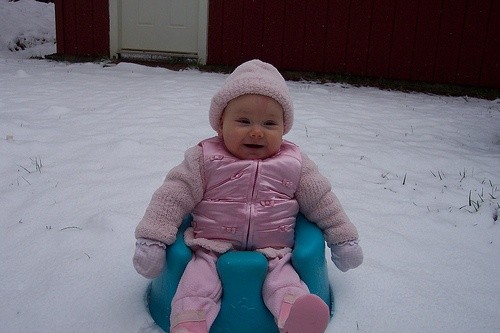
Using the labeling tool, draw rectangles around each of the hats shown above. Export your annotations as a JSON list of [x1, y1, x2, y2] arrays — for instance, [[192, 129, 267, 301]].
[[209, 59, 295, 134]]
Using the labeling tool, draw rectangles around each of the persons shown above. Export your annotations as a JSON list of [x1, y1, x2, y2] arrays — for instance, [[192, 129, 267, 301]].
[[133, 58, 363, 333]]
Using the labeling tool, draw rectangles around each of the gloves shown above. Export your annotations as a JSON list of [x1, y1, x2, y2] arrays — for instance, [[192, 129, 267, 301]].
[[131, 237, 167, 279], [330, 242, 363, 273]]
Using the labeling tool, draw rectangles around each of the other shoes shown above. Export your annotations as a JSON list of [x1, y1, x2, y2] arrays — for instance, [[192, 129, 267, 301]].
[[283, 293, 330, 333]]
